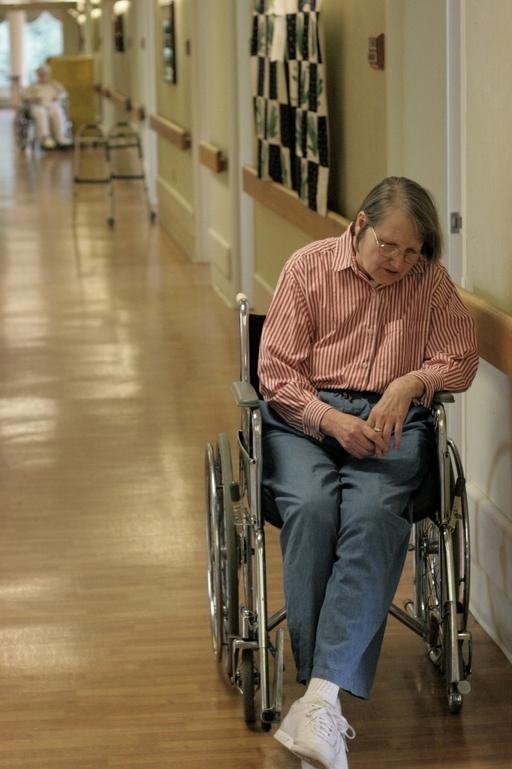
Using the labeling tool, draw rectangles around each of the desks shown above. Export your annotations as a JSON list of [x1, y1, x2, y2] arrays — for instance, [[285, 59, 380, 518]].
[[49, 55, 96, 133]]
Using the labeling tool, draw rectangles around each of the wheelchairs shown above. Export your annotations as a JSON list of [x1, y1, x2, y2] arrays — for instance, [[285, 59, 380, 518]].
[[13, 98, 73, 149], [203, 291, 472, 732]]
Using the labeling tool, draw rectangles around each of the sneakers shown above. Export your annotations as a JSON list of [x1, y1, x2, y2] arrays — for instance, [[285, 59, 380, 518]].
[[273, 692, 349, 768]]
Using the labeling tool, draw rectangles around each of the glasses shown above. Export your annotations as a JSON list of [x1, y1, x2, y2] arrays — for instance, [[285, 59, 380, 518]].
[[370, 224, 420, 265]]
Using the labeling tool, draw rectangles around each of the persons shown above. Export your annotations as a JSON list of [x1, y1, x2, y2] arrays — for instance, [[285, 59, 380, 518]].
[[257, 176, 482, 769], [24, 64, 73, 148]]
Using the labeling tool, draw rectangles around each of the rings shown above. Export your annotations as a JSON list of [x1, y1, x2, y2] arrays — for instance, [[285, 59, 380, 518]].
[[373, 426, 383, 432]]
